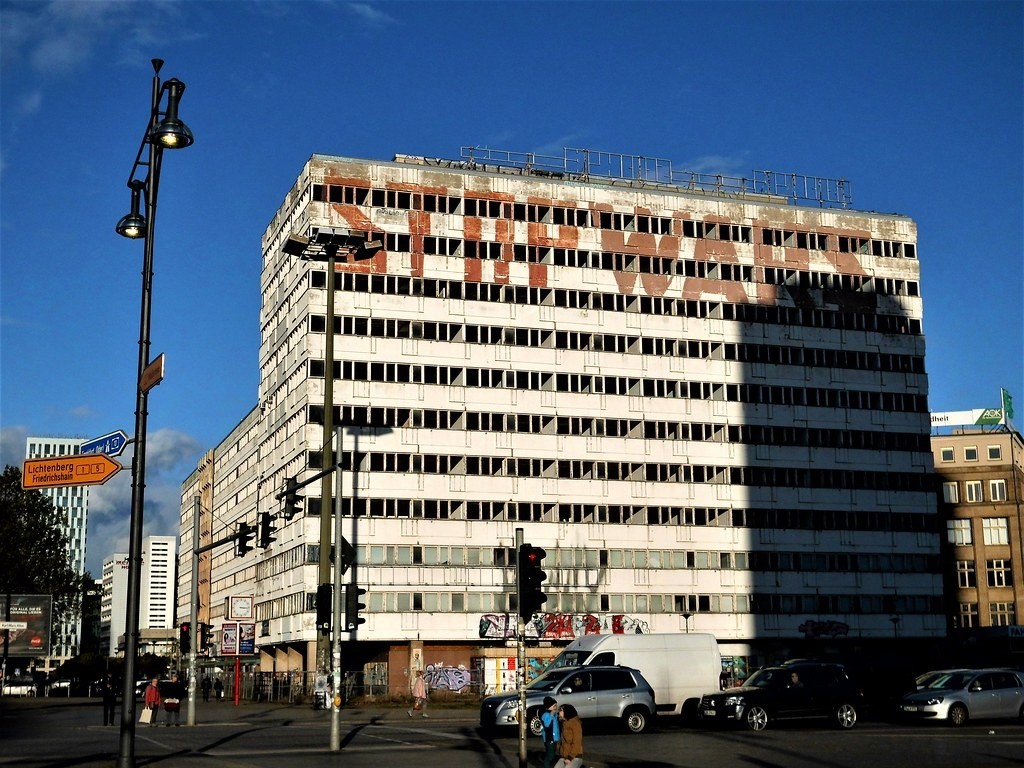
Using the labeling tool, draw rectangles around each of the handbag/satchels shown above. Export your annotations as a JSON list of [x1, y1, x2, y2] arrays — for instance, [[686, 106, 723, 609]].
[[138, 707, 153, 724], [221, 689, 224, 697], [413, 698, 421, 710]]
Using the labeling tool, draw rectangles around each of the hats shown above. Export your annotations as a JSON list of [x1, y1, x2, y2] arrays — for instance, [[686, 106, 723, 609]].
[[543, 697, 557, 709]]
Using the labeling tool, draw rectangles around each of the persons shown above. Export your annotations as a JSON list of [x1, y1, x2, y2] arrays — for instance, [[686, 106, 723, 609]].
[[166, 675, 185, 726], [98, 667, 120, 725], [214, 678, 224, 704], [145, 676, 160, 728], [971, 680, 983, 692], [201, 675, 212, 704], [785, 673, 803, 689], [407, 671, 429, 719], [554, 705, 584, 768], [540, 696, 560, 768], [571, 676, 584, 692]]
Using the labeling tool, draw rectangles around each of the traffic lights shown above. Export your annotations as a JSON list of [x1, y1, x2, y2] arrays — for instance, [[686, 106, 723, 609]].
[[238, 521, 254, 554], [200, 622, 214, 653], [518, 543, 547, 617], [344, 584, 366, 632], [284, 478, 305, 521], [179, 622, 191, 653], [256, 512, 277, 548]]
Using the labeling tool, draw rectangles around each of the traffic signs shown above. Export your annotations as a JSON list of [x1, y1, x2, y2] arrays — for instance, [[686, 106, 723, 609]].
[[21, 430, 129, 490]]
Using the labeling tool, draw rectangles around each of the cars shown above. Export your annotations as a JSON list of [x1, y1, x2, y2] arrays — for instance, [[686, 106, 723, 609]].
[[53, 680, 71, 691], [915, 669, 957, 690], [899, 669, 1024, 728], [481, 665, 657, 735], [0, 681, 36, 697]]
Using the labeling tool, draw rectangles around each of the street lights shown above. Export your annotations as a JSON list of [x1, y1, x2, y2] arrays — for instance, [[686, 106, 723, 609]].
[[889, 618, 900, 699], [680, 612, 693, 633], [115, 77, 195, 768], [281, 225, 385, 709]]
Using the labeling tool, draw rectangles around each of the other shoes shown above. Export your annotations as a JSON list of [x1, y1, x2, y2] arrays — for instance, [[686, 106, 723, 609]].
[[407, 710, 412, 717], [175, 723, 180, 728], [422, 713, 430, 718], [166, 723, 170, 727]]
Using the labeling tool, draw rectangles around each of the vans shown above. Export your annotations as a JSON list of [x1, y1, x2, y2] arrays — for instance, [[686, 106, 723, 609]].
[[545, 633, 725, 729]]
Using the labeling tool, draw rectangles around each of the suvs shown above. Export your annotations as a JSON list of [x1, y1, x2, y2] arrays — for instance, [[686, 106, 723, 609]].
[[698, 658, 864, 730]]
